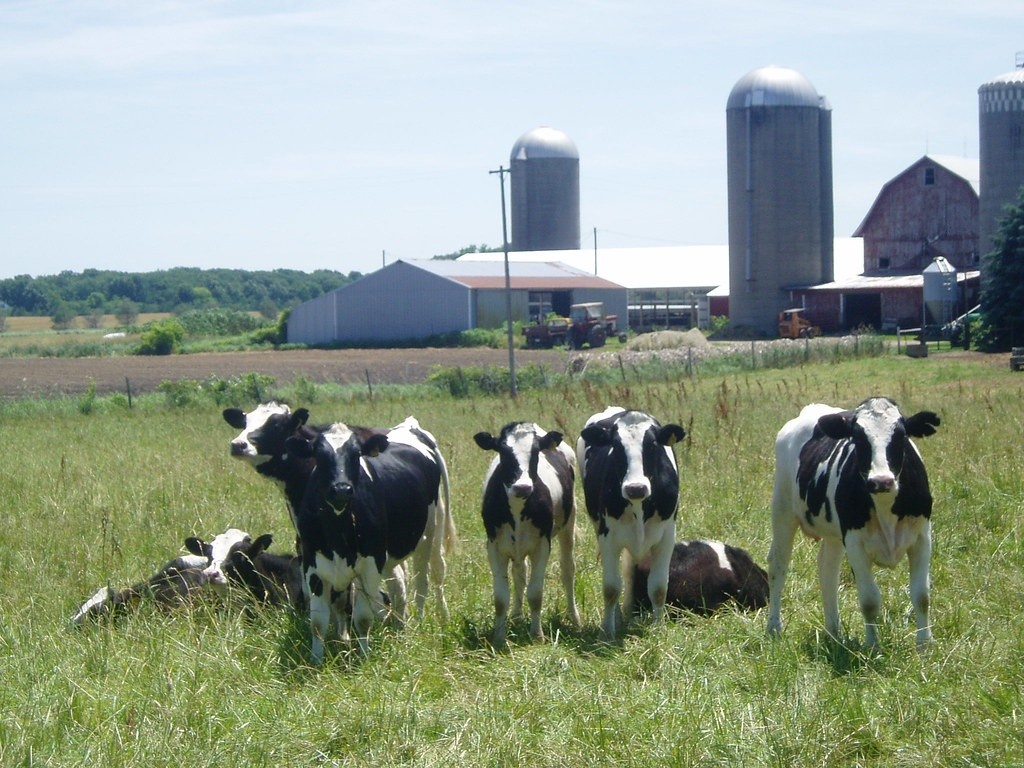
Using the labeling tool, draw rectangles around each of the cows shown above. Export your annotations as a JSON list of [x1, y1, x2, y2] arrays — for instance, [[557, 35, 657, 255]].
[[72, 396, 940, 655]]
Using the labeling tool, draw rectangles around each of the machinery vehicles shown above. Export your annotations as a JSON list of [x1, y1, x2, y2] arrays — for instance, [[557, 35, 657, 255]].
[[568, 301, 628, 350], [778, 308, 823, 340]]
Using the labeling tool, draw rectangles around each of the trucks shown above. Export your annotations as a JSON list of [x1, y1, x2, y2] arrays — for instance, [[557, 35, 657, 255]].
[[521, 318, 572, 350]]
